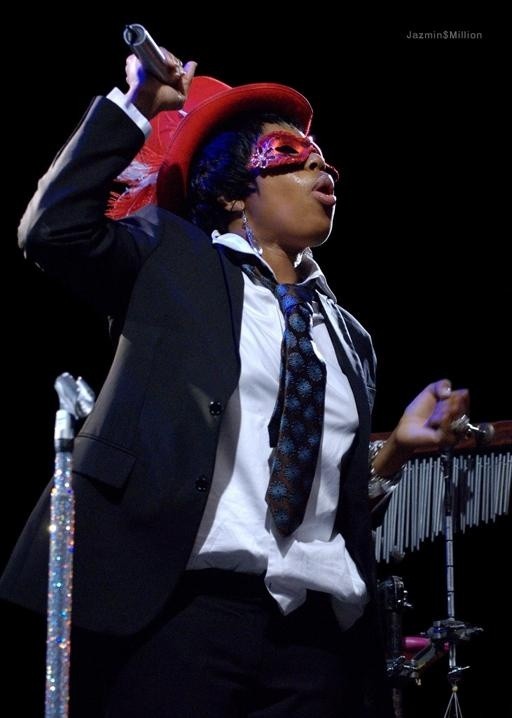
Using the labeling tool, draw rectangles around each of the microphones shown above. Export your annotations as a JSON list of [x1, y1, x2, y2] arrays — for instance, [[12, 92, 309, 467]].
[[124, 21, 184, 95]]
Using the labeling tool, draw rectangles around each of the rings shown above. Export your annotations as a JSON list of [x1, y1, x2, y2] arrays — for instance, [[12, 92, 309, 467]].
[[449, 413, 469, 434]]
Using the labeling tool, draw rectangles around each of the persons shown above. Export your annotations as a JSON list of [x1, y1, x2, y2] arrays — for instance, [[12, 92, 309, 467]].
[[15, 43, 471, 718]]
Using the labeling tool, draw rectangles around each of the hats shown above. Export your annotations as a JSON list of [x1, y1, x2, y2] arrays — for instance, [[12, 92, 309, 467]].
[[109, 63, 316, 215]]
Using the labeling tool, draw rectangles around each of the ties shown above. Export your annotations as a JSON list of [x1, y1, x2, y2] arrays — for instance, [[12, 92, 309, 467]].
[[241, 261, 331, 539]]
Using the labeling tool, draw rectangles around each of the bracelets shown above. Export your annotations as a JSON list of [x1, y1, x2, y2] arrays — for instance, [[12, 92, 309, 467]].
[[368, 438, 407, 498]]
